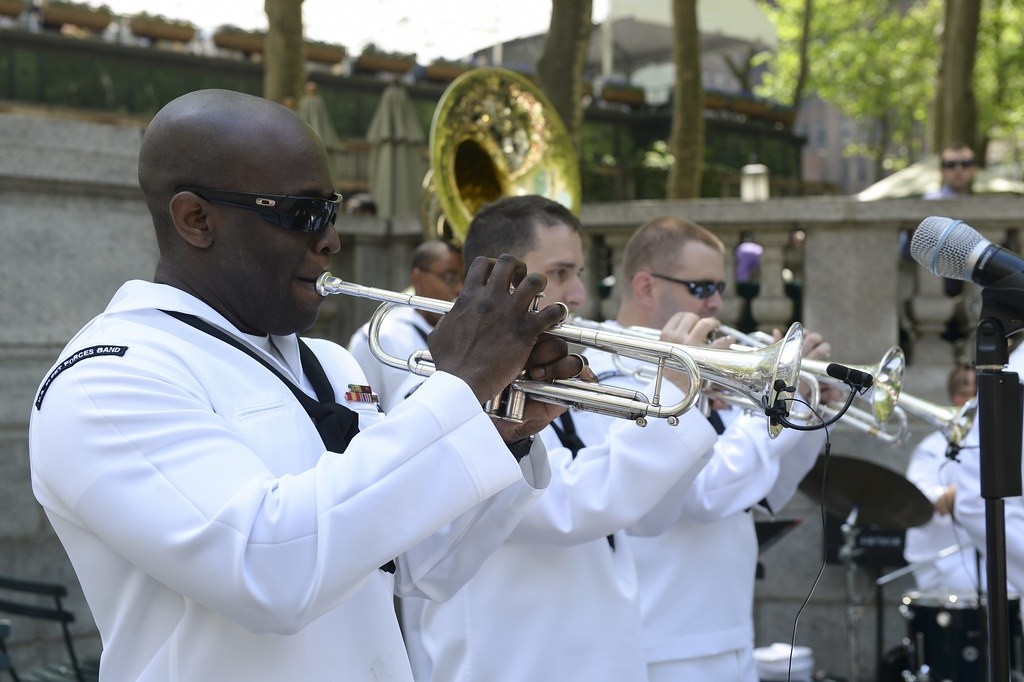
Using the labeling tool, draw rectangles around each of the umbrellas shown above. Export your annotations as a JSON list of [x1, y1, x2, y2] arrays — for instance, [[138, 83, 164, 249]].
[[364, 82, 426, 219], [295, 81, 343, 217]]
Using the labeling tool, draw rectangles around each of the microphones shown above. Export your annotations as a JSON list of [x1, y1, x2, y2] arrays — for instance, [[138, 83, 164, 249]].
[[909, 214, 1024, 289], [826, 363, 873, 388]]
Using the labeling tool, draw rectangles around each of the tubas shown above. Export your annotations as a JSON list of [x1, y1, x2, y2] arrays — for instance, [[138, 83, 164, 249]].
[[416, 62, 585, 255]]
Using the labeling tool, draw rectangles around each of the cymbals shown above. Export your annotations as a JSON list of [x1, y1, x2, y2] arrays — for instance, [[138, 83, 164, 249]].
[[795, 452, 935, 535]]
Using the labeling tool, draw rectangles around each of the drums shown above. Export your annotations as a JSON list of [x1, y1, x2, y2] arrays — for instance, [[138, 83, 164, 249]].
[[899, 577, 1022, 682]]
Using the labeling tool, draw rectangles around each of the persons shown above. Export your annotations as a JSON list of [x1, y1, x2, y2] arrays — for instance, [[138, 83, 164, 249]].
[[953, 235, 1024, 597], [349, 241, 468, 415], [28, 88, 589, 682], [549, 217, 842, 682], [898, 142, 977, 263], [392, 194, 734, 682], [904, 367, 1024, 682]]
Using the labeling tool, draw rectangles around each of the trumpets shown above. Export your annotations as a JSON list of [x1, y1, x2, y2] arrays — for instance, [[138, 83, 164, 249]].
[[315, 268, 808, 442], [556, 307, 978, 450]]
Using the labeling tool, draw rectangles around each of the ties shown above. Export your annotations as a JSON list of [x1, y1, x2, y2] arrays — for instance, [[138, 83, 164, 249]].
[[158, 306, 396, 575], [707, 409, 775, 519], [550, 407, 615, 552]]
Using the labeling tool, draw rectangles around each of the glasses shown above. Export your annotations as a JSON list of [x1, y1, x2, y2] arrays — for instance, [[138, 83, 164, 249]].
[[172, 187, 343, 234], [422, 266, 465, 284], [649, 273, 725, 301]]
